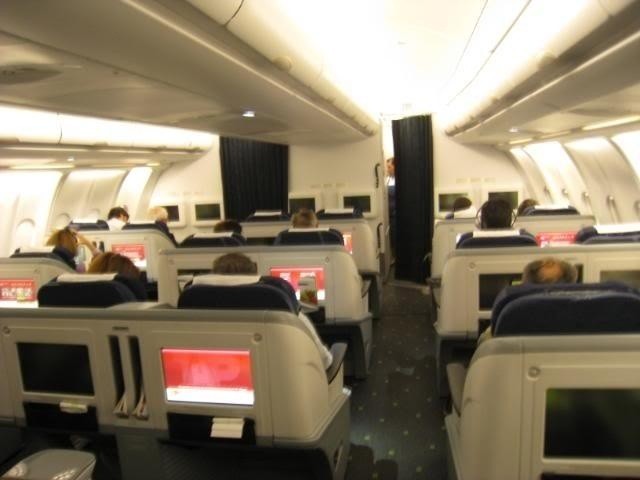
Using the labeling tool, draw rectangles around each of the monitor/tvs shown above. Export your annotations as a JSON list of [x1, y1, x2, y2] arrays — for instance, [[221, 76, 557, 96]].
[[17, 342, 94, 396], [161, 348, 254, 405], [270, 268, 325, 300], [543, 388, 640, 459], [489, 191, 518, 210], [111, 244, 146, 267], [344, 195, 371, 212], [195, 203, 221, 221], [342, 234, 353, 255], [289, 198, 315, 219], [479, 274, 522, 310], [0, 279, 35, 301], [600, 270, 640, 292], [534, 230, 576, 246], [438, 193, 468, 212], [160, 206, 179, 221], [178, 269, 213, 295]]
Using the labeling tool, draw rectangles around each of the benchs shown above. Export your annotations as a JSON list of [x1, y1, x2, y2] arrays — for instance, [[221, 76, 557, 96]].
[[0, 252, 75, 303], [445, 282, 640, 479], [432, 206, 596, 287], [1, 272, 352, 480], [425, 228, 640, 408], [69, 217, 178, 284], [157, 244, 373, 381], [240, 206, 387, 321]]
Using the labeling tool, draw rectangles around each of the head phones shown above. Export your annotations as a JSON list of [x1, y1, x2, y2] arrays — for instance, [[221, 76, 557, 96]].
[[474, 198, 518, 229]]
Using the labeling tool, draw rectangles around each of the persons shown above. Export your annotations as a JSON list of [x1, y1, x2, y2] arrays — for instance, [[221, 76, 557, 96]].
[[480, 201, 514, 229], [87, 251, 156, 298], [147, 206, 177, 242], [104, 207, 129, 231], [386, 158, 395, 260], [44, 226, 102, 272], [211, 252, 257, 274], [455, 198, 473, 212], [289, 209, 320, 227], [474, 255, 578, 345], [212, 220, 241, 232], [518, 199, 538, 216]]
